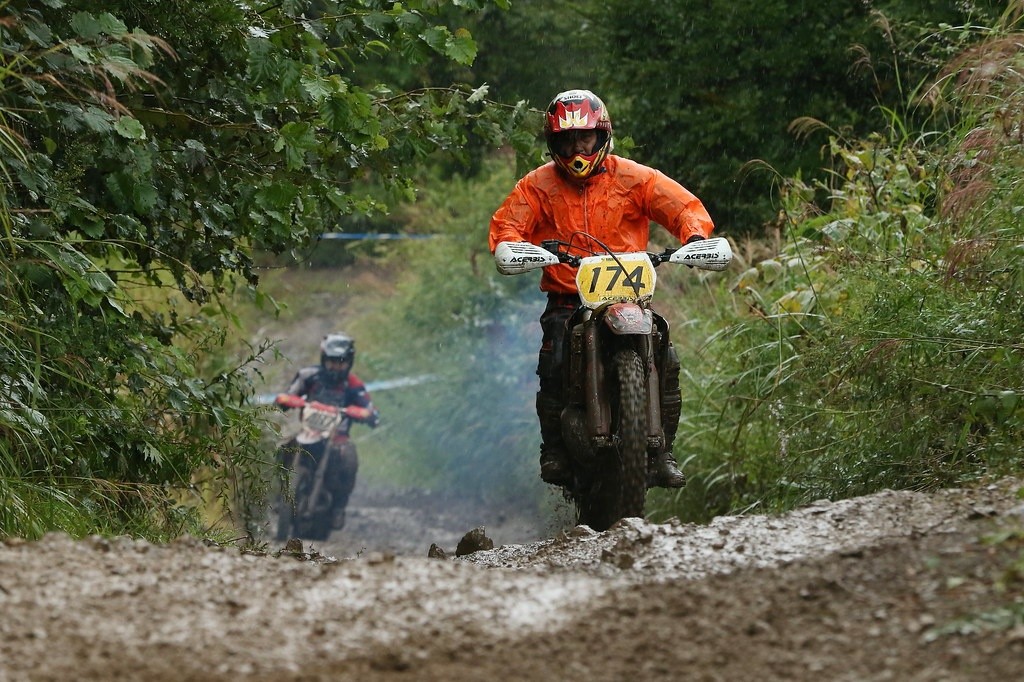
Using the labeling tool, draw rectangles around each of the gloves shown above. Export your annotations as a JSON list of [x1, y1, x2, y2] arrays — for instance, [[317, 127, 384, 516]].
[[366, 411, 379, 427], [280, 404, 287, 411], [683, 236, 704, 268]]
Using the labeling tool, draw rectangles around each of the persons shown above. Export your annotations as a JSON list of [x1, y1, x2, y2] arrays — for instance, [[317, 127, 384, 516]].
[[488, 89, 716, 489], [271, 333, 379, 529]]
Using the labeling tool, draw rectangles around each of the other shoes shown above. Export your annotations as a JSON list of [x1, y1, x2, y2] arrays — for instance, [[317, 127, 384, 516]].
[[653, 452, 686, 488], [540, 445, 567, 486]]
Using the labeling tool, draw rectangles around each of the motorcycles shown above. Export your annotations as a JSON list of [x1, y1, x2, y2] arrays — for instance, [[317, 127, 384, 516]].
[[273, 393, 371, 541], [496, 237, 734, 531]]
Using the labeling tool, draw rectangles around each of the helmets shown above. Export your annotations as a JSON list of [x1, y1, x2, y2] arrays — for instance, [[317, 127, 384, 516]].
[[544, 90, 613, 181], [320, 333, 355, 385]]
